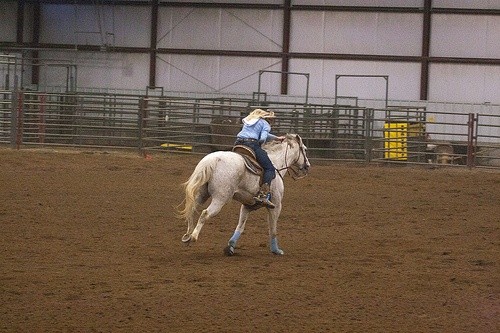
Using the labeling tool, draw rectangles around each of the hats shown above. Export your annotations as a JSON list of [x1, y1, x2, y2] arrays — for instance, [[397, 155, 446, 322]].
[[242, 108, 277, 127]]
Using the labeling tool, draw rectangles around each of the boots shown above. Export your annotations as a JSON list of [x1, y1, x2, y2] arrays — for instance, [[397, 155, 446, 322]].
[[256, 183, 275, 209]]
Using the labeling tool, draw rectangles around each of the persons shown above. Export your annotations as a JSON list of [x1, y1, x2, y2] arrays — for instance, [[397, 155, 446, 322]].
[[233, 108, 277, 209]]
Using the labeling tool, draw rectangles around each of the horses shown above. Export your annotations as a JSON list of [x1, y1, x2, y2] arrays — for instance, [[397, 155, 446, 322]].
[[169, 133, 311, 257]]
